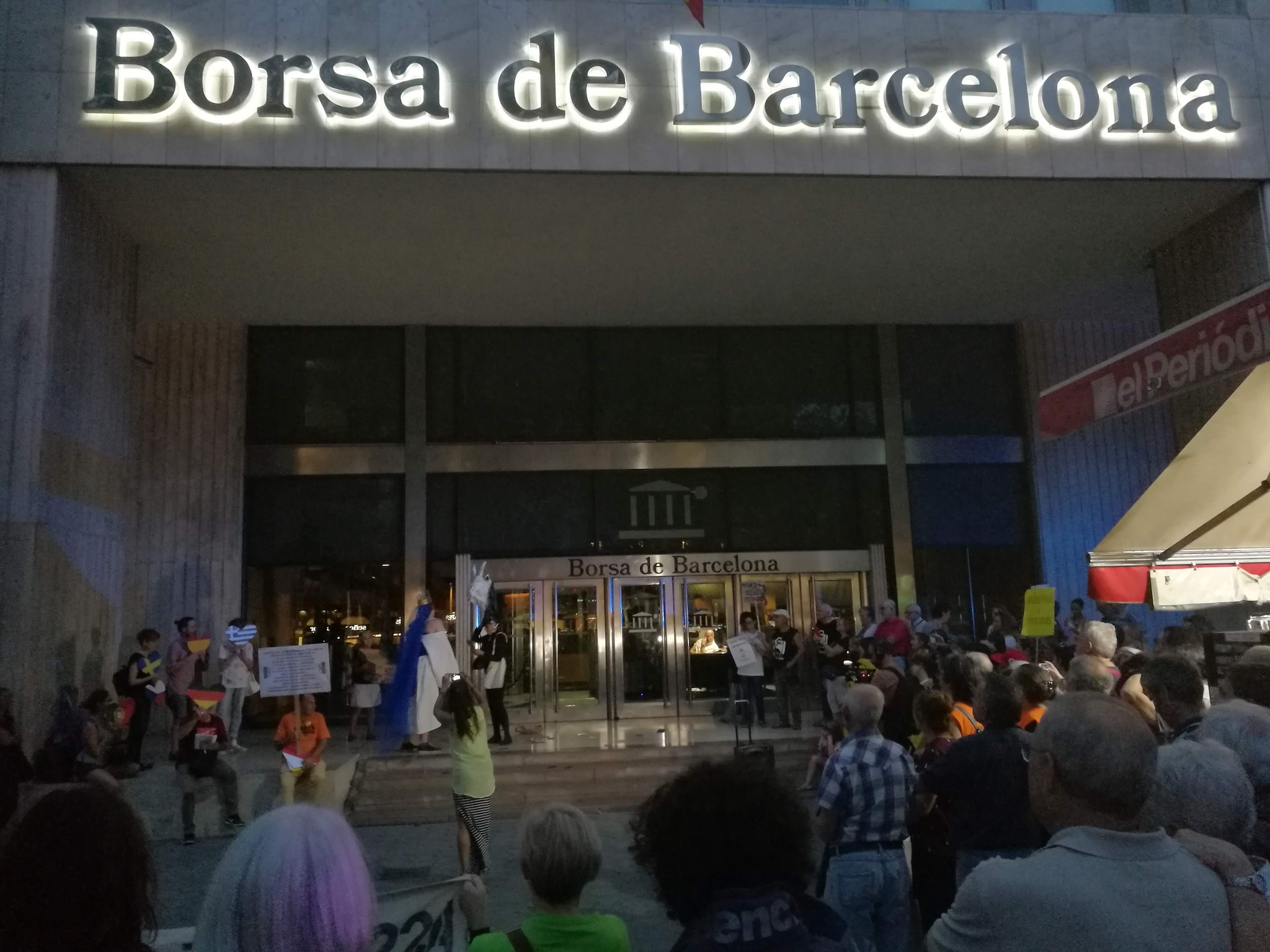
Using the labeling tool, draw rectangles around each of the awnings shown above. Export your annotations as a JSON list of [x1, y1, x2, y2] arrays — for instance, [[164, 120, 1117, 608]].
[[1085, 361, 1270, 614]]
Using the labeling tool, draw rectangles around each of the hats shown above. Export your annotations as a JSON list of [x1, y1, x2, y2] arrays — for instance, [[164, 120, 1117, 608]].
[[772, 609, 790, 620]]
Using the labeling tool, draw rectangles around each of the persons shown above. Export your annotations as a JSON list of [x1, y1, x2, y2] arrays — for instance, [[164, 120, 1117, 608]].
[[467, 803, 631, 952], [254, 686, 275, 686], [626, 599, 1270, 951], [347, 630, 382, 741], [166, 617, 206, 760], [471, 609, 513, 746], [0, 686, 28, 828], [73, 689, 157, 843], [171, 704, 245, 845], [126, 629, 161, 770], [434, 671, 495, 877], [213, 617, 274, 754], [273, 694, 331, 804], [400, 590, 459, 755]]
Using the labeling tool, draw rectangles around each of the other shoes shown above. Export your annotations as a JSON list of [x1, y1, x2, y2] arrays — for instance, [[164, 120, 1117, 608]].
[[417, 742, 442, 753], [759, 721, 769, 728], [182, 834, 195, 845], [793, 725, 802, 730], [224, 814, 245, 826], [740, 720, 756, 727], [812, 720, 824, 726], [168, 751, 178, 760], [366, 733, 377, 740], [500, 738, 512, 745], [795, 784, 812, 792], [347, 734, 357, 741], [488, 736, 501, 743], [771, 722, 791, 728], [228, 745, 247, 753], [401, 742, 419, 752]]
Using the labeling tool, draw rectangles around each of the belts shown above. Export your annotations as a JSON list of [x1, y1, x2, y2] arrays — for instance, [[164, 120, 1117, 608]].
[[829, 840, 903, 857]]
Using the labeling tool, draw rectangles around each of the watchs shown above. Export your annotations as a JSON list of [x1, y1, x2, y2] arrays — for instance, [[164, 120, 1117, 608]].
[[464, 926, 491, 944]]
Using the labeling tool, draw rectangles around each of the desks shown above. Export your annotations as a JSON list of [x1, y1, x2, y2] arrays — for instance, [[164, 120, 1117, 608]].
[[693, 651, 731, 697]]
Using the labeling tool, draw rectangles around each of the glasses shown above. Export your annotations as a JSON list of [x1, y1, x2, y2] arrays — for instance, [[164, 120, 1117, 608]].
[[904, 612, 915, 615]]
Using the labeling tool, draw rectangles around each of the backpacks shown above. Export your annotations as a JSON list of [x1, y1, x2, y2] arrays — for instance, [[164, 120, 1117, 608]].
[[111, 665, 130, 697]]
